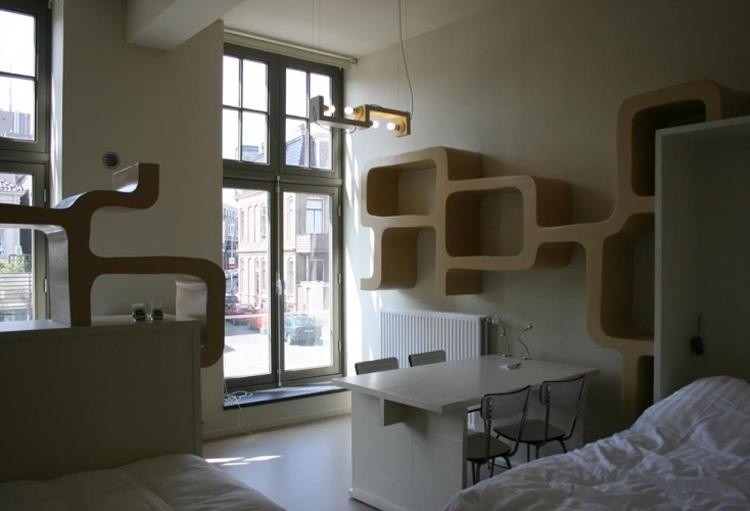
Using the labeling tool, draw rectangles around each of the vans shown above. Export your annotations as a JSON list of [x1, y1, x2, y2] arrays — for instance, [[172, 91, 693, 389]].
[[224, 293, 240, 307]]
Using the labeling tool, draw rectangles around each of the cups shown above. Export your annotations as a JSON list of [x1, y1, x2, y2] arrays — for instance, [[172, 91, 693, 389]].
[[128, 303, 146, 321], [147, 302, 163, 320]]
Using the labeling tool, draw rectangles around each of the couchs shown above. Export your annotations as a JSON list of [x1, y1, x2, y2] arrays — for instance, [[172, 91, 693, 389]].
[[2, 318, 283, 511]]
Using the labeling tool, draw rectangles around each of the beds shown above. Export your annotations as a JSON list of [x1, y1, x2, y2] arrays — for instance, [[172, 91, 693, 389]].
[[445, 375, 750, 511]]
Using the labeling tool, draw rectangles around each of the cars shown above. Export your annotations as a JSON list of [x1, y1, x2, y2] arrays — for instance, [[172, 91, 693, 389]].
[[247, 308, 270, 331], [284, 316, 322, 344], [224, 303, 251, 324]]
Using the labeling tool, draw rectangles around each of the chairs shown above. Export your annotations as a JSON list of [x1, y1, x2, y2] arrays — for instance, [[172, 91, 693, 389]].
[[493, 376, 585, 464], [408, 351, 445, 368], [354, 358, 399, 376], [466, 385, 533, 487]]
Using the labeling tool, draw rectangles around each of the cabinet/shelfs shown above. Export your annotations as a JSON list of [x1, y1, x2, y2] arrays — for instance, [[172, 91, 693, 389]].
[[358, 79, 747, 434]]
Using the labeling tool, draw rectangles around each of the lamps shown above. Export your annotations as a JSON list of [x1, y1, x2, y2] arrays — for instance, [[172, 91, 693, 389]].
[[308, 1, 409, 136], [492, 314, 512, 358], [519, 324, 534, 361]]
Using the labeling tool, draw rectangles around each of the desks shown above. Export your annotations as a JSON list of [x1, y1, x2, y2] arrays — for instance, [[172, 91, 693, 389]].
[[332, 353, 598, 510]]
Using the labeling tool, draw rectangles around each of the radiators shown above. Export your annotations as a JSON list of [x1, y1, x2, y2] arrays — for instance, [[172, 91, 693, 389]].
[[377, 306, 489, 369]]
